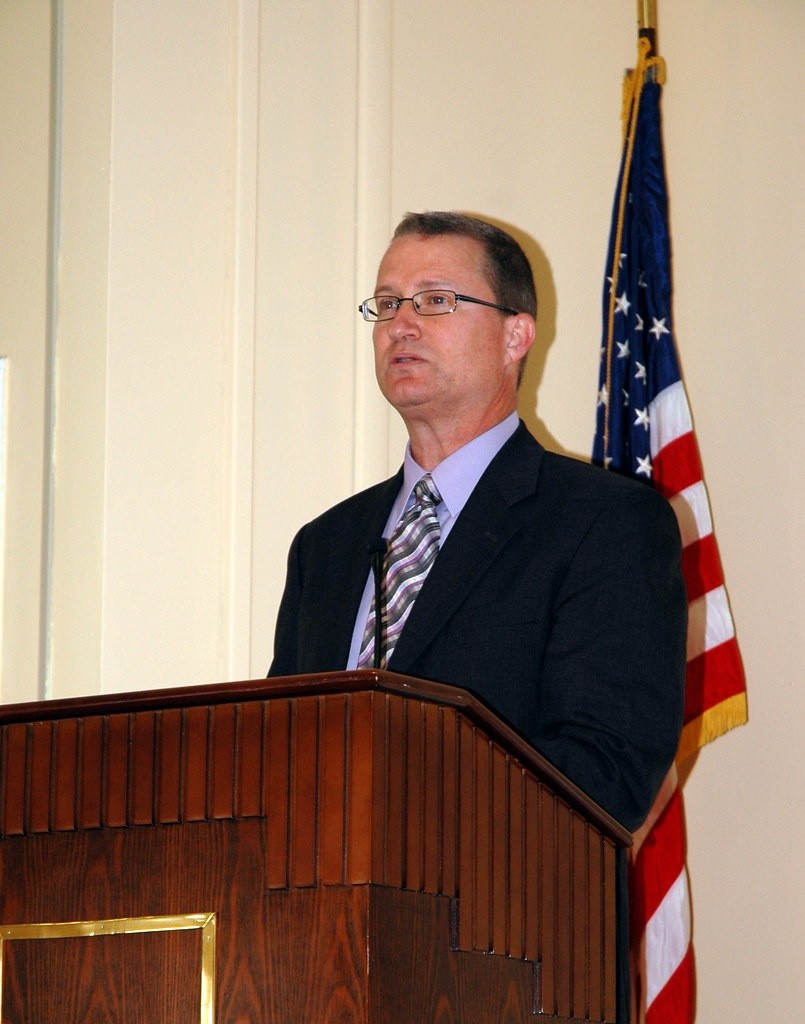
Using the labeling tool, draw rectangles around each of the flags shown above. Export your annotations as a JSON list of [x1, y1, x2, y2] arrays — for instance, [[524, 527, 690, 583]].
[[589, 28, 749, 1024]]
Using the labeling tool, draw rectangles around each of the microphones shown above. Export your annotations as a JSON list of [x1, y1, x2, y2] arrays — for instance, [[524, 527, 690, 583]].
[[361, 538, 388, 669]]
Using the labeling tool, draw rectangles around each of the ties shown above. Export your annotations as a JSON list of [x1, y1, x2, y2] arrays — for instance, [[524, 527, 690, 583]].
[[355, 476, 444, 673]]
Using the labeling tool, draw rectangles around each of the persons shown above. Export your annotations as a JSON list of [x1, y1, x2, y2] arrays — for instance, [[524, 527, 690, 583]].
[[267, 212, 688, 1024]]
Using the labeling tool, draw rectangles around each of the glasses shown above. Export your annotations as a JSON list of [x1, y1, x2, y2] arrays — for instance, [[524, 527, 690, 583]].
[[358, 290, 520, 322]]
